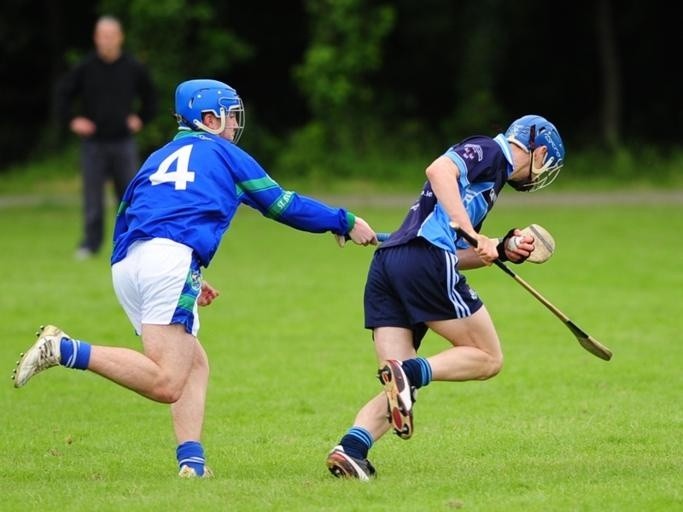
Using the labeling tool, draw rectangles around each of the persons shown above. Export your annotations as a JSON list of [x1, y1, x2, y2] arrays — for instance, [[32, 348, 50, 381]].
[[50, 15, 158, 262], [324, 114, 567, 479], [11, 78, 377, 478]]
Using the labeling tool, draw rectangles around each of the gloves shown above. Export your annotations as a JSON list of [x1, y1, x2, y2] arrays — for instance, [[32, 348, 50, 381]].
[[496, 224, 537, 268]]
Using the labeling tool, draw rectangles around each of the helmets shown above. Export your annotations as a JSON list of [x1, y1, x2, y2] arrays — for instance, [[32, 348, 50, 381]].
[[502, 110, 569, 173], [172, 76, 244, 132]]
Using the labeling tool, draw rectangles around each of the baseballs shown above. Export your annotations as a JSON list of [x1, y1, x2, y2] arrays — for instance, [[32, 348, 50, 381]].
[[505, 232, 529, 260]]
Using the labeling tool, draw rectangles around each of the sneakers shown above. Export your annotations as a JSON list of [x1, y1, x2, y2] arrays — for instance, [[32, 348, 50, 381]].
[[7, 320, 71, 390], [176, 459, 218, 482], [326, 441, 379, 485], [374, 357, 419, 442]]
